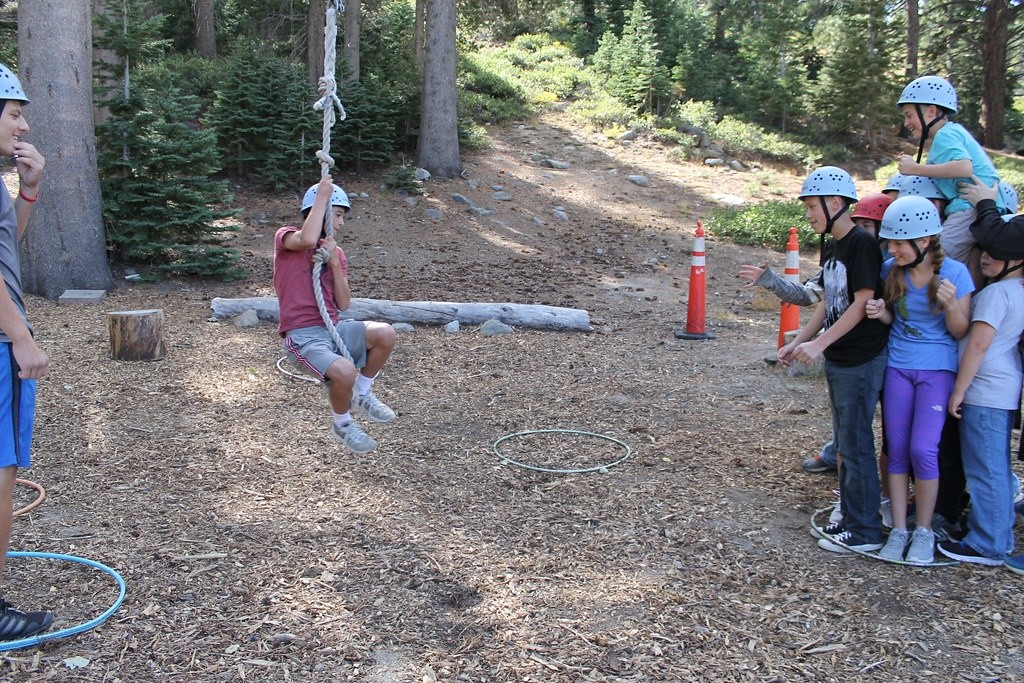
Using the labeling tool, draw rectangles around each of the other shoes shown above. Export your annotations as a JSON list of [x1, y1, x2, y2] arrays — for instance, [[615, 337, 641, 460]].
[[906, 511, 960, 534]]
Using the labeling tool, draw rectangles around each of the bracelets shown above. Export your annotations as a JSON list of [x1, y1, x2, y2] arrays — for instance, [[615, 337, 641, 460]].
[[19, 185, 38, 201]]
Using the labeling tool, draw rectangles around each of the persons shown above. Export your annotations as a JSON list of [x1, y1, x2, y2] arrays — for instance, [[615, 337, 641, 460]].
[[0, 62, 52, 637], [739, 76, 1024, 577], [275, 175, 397, 454]]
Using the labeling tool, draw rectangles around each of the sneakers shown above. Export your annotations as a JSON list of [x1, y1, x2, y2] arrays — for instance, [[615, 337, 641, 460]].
[[1003, 553, 1024, 575], [932, 520, 960, 541], [817, 530, 884, 553], [802, 454, 830, 472], [1013, 501, 1024, 517], [905, 527, 935, 564], [936, 540, 1004, 566], [829, 488, 843, 523], [351, 387, 395, 423], [948, 529, 1013, 553], [0, 598, 55, 640], [879, 528, 909, 561], [810, 521, 849, 539], [331, 419, 378, 454], [878, 492, 893, 527]]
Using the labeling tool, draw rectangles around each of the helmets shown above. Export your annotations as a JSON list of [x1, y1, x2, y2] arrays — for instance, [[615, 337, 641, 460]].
[[0, 62, 30, 106], [896, 76, 959, 117], [797, 165, 859, 204], [878, 195, 943, 240], [881, 173, 909, 193], [851, 192, 895, 222], [301, 182, 350, 214], [995, 180, 1018, 214], [898, 175, 947, 199], [1000, 212, 1019, 224]]
